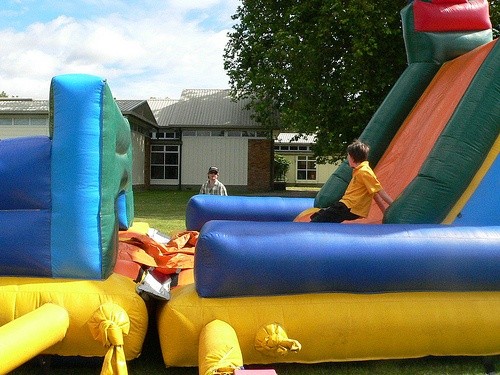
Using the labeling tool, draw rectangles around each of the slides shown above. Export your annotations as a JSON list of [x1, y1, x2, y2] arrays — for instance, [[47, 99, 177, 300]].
[[292, 36, 500, 228]]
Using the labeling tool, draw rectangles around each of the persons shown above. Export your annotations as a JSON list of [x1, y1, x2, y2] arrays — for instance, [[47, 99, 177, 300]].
[[199, 165, 229, 197], [309, 139, 394, 224]]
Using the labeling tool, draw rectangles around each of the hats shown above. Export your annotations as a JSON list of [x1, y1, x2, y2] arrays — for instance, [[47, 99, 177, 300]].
[[209, 166, 218, 172]]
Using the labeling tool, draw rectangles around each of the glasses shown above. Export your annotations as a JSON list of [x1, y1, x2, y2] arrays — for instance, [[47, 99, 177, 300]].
[[209, 171, 218, 175]]
